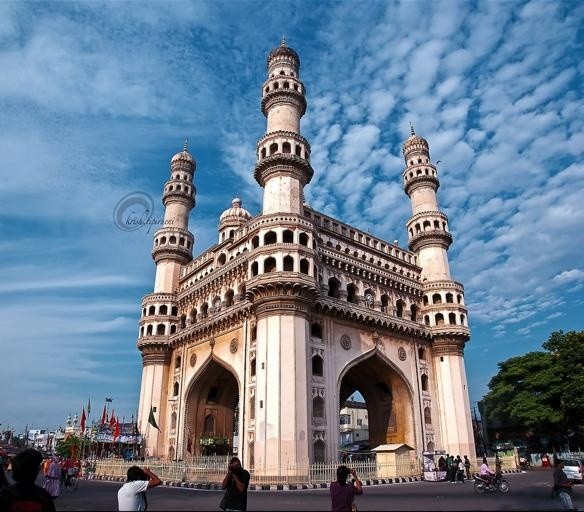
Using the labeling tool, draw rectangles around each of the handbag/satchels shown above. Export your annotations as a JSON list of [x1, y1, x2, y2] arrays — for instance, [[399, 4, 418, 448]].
[[220, 496, 243, 511]]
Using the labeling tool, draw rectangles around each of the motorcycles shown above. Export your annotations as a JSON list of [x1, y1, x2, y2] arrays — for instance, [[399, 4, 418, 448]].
[[472, 473, 509, 495], [61, 467, 79, 490]]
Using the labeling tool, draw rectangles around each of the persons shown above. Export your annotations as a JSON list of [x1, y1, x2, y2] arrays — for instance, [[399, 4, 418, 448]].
[[552, 458, 574, 509], [329, 465, 363, 511], [495, 455, 502, 473], [438, 452, 472, 485], [218, 456, 249, 512], [519, 456, 528, 466], [479, 458, 498, 492], [0, 448, 56, 511], [116, 464, 161, 511], [340, 451, 369, 464], [41, 455, 96, 498]]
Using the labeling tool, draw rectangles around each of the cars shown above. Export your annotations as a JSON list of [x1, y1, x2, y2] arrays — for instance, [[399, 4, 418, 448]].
[[562, 458, 584, 481]]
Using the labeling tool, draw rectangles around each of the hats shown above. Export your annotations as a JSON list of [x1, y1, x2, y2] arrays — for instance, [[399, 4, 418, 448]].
[[12, 448, 43, 472]]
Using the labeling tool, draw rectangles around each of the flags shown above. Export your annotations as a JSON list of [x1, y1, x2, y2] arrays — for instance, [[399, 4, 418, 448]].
[[121, 415, 126, 441], [80, 404, 86, 433], [112, 415, 119, 441], [87, 394, 91, 417], [99, 402, 106, 429], [108, 407, 116, 428], [128, 413, 135, 440]]
